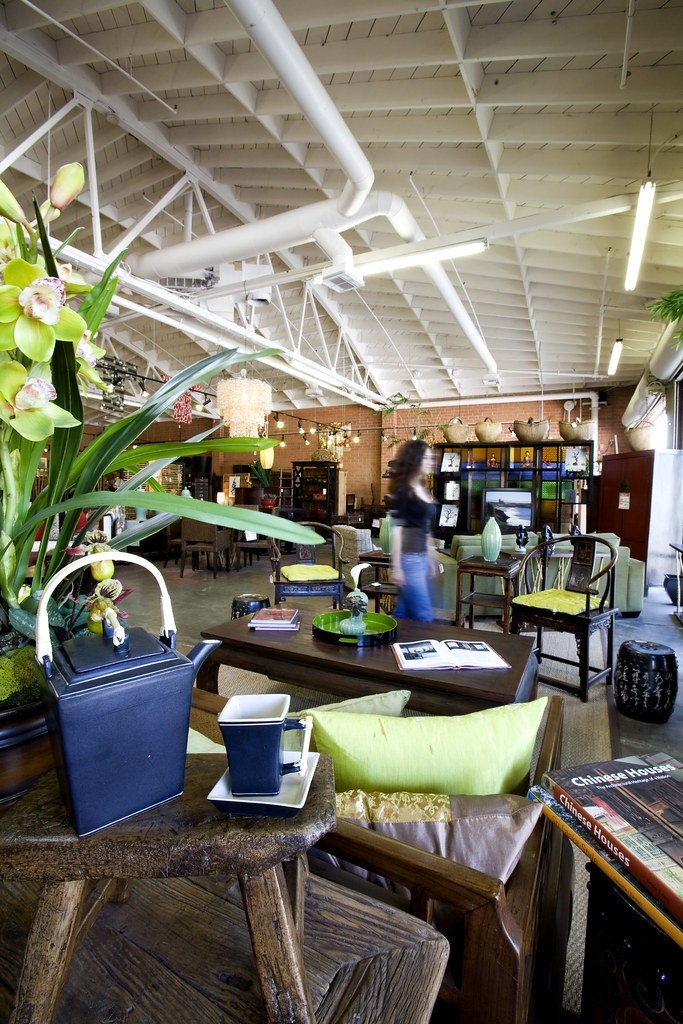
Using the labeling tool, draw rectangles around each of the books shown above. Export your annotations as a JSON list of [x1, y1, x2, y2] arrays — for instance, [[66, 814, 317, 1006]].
[[528, 752, 683, 951], [390, 639, 512, 671], [247, 608, 301, 631]]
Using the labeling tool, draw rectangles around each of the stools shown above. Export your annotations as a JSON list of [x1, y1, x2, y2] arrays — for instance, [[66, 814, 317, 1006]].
[[0, 745, 335, 1024], [614, 637, 678, 722]]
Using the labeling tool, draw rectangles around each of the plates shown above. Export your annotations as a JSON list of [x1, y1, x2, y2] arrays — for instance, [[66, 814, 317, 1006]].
[[208, 751, 321, 818]]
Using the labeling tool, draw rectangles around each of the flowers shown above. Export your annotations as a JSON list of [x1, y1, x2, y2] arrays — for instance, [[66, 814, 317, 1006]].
[[0, 154, 327, 712]]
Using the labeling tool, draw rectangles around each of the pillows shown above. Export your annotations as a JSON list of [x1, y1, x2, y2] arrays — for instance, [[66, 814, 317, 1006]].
[[255, 689, 551, 904]]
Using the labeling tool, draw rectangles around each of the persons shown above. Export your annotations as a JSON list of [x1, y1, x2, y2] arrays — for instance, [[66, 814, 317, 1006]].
[[385, 440, 436, 623], [520, 451, 532, 468], [444, 506, 450, 523], [300, 545, 309, 559], [95, 578, 130, 655], [487, 453, 498, 468], [447, 454, 456, 468], [573, 451, 581, 463], [231, 477, 236, 494]]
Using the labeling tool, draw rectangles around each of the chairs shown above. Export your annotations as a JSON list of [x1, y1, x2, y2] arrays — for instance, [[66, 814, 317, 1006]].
[[273, 520, 349, 610], [506, 537, 620, 702], [164, 501, 280, 578]]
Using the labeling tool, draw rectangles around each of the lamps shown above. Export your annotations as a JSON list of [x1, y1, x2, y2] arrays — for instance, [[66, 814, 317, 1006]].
[[109, 372, 428, 450], [607, 321, 623, 376], [216, 287, 274, 438], [616, 104, 659, 289]]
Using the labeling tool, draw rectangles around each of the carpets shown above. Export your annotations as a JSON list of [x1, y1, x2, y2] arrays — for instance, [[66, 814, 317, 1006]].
[[194, 596, 619, 1016]]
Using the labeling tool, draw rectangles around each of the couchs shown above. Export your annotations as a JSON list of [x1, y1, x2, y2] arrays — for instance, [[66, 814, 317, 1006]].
[[186, 686, 563, 1024], [430, 531, 648, 619]]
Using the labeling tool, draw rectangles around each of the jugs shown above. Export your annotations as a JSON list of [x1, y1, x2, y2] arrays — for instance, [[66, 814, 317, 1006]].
[[35, 552, 222, 839]]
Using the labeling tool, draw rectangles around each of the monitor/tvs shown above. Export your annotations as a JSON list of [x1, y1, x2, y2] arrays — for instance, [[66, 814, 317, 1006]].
[[482, 488, 536, 535]]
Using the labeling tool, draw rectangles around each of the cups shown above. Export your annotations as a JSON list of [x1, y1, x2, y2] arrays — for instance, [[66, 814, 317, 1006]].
[[218, 693, 313, 796]]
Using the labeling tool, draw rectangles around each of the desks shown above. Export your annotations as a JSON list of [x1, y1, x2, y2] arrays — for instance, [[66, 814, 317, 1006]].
[[0, 855, 452, 1024]]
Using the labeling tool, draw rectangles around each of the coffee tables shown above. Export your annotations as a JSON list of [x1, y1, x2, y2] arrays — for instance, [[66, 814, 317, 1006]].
[[196, 602, 540, 734]]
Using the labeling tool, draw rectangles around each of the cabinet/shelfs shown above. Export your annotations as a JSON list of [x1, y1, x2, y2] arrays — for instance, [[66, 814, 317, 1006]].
[[356, 549, 399, 616], [456, 553, 525, 633], [432, 440, 595, 539], [191, 459, 348, 522], [597, 448, 682, 588]]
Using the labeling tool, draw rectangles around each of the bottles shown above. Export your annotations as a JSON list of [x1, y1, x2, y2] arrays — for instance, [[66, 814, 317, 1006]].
[[481, 516, 502, 562]]
